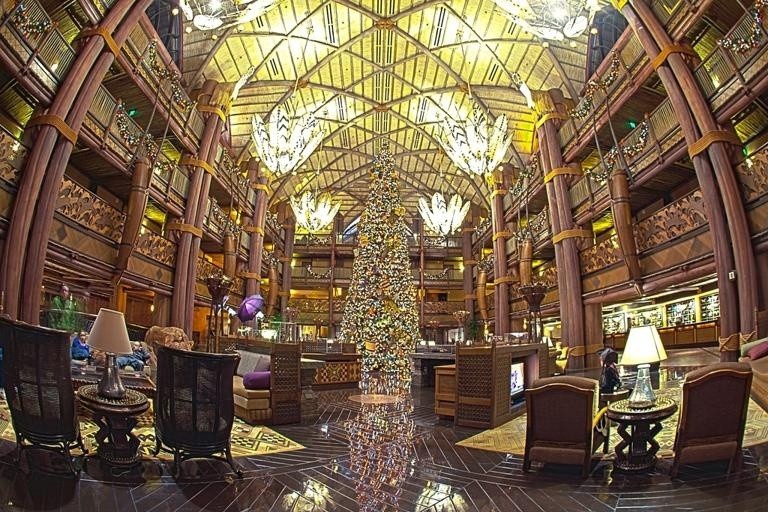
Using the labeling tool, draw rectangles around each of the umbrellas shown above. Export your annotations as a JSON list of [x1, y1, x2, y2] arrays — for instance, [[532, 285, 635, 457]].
[[237, 294, 265, 323]]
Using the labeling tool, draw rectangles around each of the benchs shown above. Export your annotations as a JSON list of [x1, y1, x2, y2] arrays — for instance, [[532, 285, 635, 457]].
[[43, 309, 151, 379], [218, 335, 301, 426]]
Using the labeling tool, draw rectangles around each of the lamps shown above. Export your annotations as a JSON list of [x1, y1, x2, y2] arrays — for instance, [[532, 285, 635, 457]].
[[497, 0, 595, 43], [248, 26, 328, 176], [86, 309, 134, 399], [434, 30, 517, 179], [619, 325, 668, 410], [178, 1, 280, 34], [288, 109, 342, 233], [416, 117, 472, 236]]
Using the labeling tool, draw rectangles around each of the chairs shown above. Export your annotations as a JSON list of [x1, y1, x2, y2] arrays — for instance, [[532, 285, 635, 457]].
[[670, 362, 753, 478], [1, 313, 90, 483], [152, 340, 244, 484], [521, 376, 611, 479]]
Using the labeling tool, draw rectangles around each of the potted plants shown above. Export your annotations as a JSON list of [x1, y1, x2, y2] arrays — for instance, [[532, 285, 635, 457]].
[[464, 319, 482, 347]]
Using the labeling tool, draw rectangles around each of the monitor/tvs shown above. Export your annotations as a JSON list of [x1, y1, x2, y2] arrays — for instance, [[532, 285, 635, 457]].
[[511, 356, 529, 406]]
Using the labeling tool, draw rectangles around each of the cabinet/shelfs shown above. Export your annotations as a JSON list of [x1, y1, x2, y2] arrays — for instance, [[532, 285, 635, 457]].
[[409, 353, 456, 387], [433, 364, 456, 420], [454, 339, 549, 430]]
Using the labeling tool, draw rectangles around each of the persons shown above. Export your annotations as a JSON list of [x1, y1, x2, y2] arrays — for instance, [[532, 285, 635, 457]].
[[71, 329, 91, 365], [116, 341, 151, 371], [48, 285, 76, 335]]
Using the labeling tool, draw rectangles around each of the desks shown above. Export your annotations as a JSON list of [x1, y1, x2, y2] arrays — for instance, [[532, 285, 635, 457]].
[[75, 384, 151, 480], [606, 396, 678, 486], [71, 375, 157, 399]]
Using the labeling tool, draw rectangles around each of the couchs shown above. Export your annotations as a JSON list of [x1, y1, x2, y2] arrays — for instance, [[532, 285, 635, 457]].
[[738, 337, 768, 410]]
